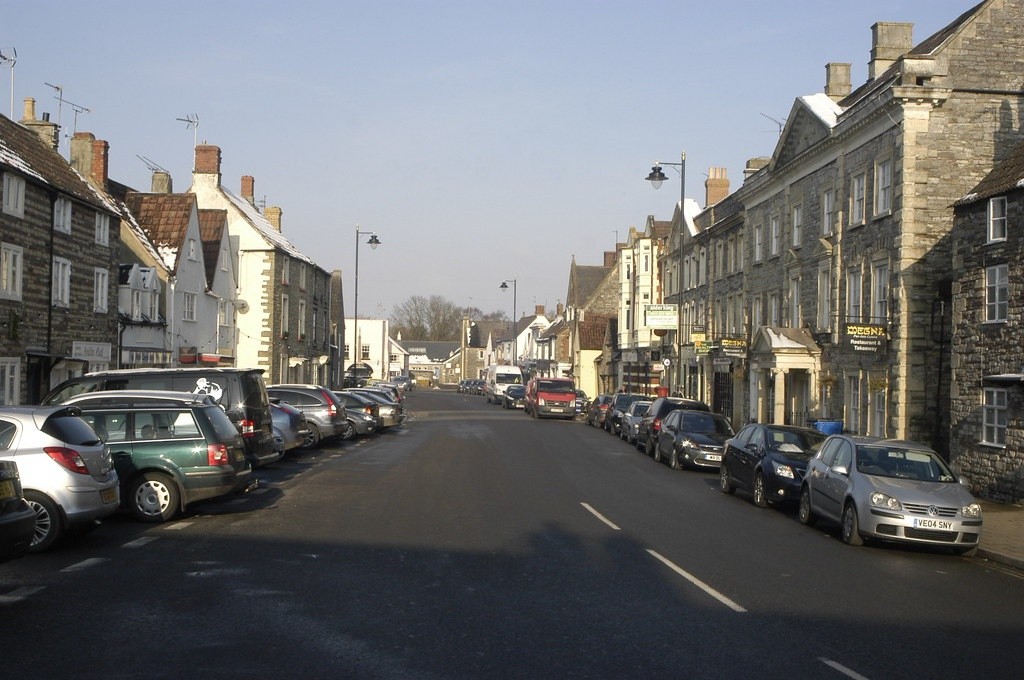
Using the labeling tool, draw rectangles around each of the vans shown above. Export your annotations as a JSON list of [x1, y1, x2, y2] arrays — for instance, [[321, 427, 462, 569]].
[[524, 377, 576, 421], [40, 366, 280, 494], [486, 364, 523, 405]]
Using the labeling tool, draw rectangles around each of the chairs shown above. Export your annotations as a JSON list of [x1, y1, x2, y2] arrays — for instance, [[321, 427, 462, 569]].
[[858, 456, 887, 475]]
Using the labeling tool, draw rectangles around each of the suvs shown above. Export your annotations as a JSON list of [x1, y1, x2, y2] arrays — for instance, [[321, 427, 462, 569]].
[[392, 376, 410, 391], [266, 384, 348, 450], [604, 393, 650, 434]]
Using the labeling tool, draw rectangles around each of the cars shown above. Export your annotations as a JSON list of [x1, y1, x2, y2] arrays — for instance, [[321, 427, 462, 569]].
[[269, 401, 310, 458], [353, 231, 381, 387], [0, 405, 121, 554], [636, 396, 710, 455], [798, 434, 982, 556], [331, 378, 405, 432], [457, 365, 591, 420], [653, 409, 737, 470], [586, 395, 614, 427], [0, 461, 37, 560], [621, 400, 653, 443], [336, 408, 377, 440], [60, 390, 253, 522], [720, 423, 829, 507]]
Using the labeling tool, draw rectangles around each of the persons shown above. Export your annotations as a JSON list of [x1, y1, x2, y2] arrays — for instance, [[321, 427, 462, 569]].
[[141, 425, 157, 440]]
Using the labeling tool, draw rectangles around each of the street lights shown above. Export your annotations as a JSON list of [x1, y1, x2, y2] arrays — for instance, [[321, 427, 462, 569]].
[[644, 162, 684, 396], [500, 280, 517, 365]]
